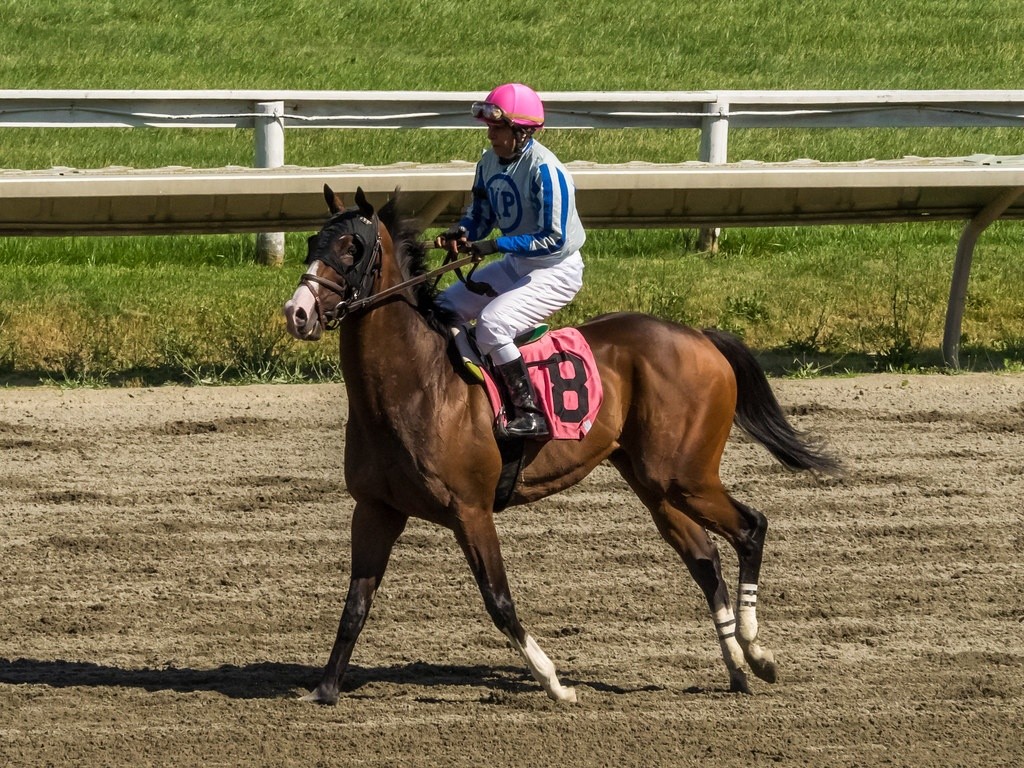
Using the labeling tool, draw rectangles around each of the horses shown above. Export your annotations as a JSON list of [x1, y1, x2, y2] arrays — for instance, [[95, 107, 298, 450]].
[[284, 182, 854, 707]]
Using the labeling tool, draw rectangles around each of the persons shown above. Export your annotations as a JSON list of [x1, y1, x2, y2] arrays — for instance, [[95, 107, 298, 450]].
[[435, 82, 586, 436]]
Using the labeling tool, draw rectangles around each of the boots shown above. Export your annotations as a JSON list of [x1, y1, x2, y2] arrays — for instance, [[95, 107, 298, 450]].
[[490, 352, 549, 439]]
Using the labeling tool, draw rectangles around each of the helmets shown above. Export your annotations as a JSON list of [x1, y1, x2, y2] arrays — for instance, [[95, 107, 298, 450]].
[[480, 83, 544, 126]]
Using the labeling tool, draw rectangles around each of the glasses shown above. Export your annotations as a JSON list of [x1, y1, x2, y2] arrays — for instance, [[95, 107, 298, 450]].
[[470, 101, 503, 121]]
